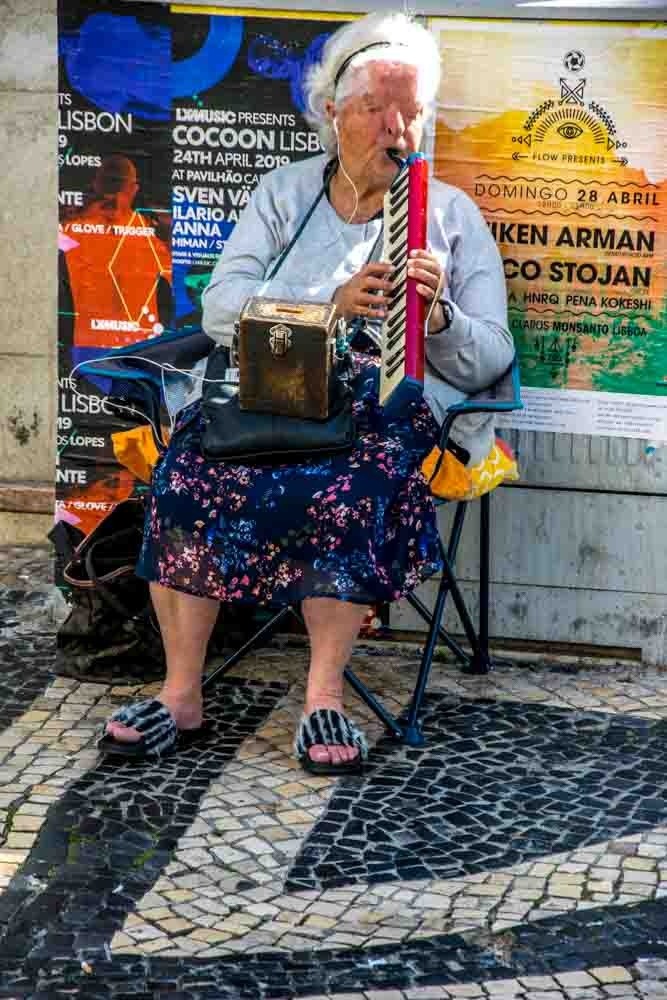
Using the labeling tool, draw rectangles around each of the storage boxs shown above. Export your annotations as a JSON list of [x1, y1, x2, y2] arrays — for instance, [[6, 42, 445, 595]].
[[239, 297, 338, 420]]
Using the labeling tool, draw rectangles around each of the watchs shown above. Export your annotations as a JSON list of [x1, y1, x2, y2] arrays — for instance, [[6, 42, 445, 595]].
[[428, 302, 454, 335]]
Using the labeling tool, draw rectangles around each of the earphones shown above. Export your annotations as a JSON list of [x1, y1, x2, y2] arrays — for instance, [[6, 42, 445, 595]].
[[333, 116, 339, 136]]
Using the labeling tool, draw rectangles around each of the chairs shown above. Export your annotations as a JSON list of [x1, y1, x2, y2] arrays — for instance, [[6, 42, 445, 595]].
[[79, 325, 524, 747]]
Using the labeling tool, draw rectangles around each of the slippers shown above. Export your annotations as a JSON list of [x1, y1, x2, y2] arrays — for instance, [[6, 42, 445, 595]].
[[96, 697, 208, 757], [293, 706, 369, 774]]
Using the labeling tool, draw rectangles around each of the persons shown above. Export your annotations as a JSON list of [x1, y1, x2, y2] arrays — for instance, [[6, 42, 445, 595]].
[[60, 153, 173, 392], [96, 13, 518, 775]]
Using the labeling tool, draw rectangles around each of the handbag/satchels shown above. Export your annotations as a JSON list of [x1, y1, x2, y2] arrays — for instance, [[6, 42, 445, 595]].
[[51, 499, 168, 687], [201, 372, 357, 463]]
